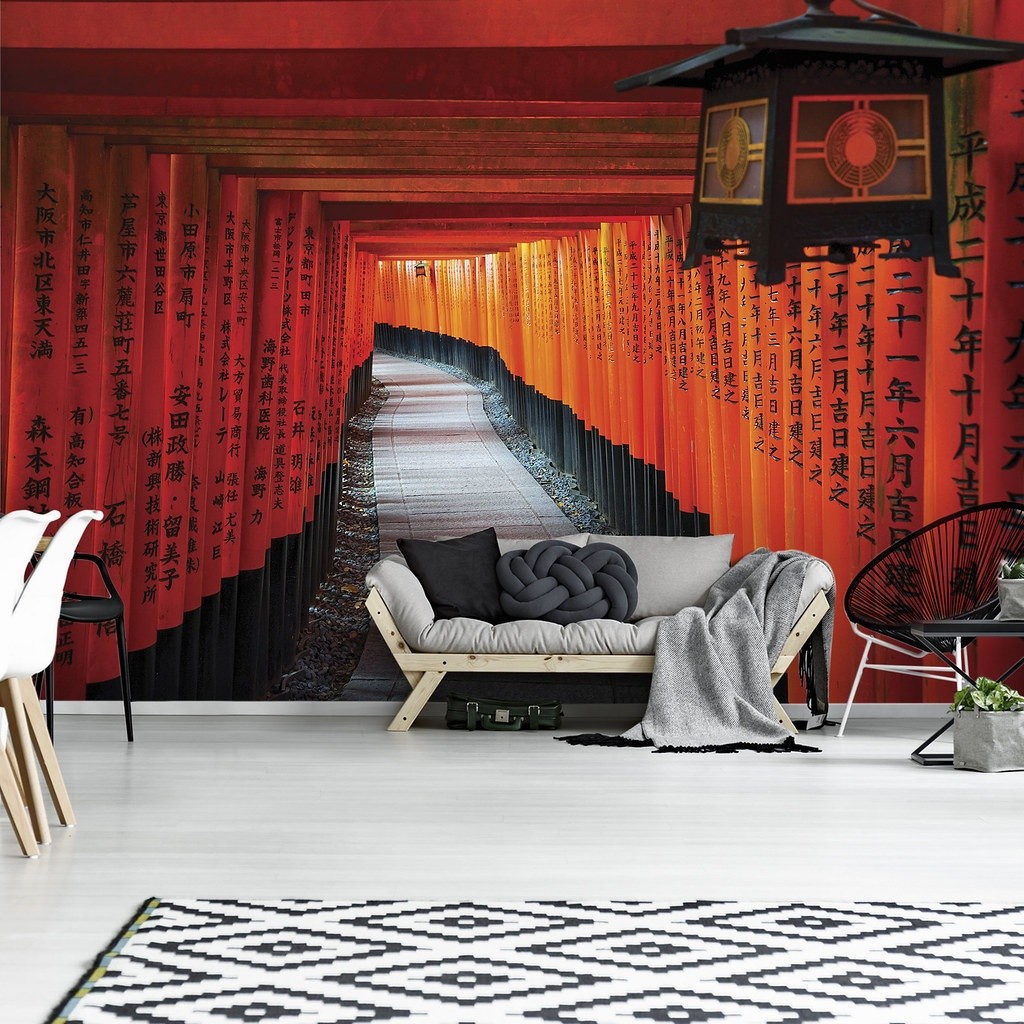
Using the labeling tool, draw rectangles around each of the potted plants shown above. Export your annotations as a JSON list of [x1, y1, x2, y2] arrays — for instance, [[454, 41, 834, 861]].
[[994, 558, 1024, 621], [946, 676, 1024, 773]]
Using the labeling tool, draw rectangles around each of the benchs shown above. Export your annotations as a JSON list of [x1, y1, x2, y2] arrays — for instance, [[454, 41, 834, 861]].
[[364, 533, 835, 731]]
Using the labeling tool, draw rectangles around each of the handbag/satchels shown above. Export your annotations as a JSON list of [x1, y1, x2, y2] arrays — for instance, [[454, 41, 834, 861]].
[[954, 686, 1024, 772], [994, 560, 1024, 621], [445, 692, 565, 731]]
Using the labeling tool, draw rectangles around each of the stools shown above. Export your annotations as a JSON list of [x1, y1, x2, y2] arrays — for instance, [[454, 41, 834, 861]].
[[30, 551, 134, 748]]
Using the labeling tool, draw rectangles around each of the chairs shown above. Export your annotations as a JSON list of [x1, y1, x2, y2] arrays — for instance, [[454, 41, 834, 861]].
[[0, 509, 104, 860], [835, 501, 1024, 738]]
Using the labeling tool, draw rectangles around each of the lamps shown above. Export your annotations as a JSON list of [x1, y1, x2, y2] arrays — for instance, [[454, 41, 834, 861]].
[[613, 0, 1024, 284], [414, 260, 432, 277]]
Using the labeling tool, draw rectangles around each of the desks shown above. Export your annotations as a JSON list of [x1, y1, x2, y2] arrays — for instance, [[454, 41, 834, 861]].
[[909, 619, 1024, 766]]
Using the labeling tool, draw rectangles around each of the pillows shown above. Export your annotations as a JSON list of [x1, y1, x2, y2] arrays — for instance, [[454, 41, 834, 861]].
[[397, 527, 511, 626], [496, 540, 639, 625]]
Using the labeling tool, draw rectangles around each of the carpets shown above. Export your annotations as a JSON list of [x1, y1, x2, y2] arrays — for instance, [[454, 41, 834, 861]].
[[43, 897, 1024, 1024]]
